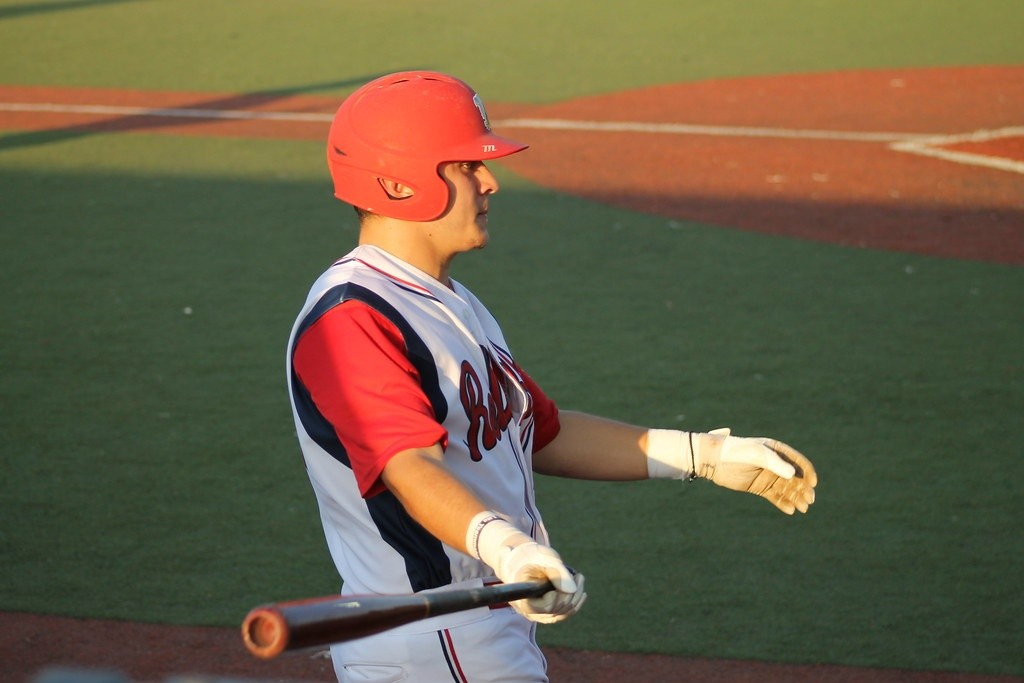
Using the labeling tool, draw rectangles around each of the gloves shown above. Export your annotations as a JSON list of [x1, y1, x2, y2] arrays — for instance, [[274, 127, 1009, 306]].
[[648, 427, 817, 515], [466, 511, 587, 624]]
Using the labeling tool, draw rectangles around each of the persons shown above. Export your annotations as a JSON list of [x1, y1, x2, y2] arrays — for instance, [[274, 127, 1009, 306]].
[[286, 74, 818, 683]]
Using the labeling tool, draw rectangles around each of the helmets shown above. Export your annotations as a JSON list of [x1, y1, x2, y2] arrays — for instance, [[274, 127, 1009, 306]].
[[327, 70, 529, 221]]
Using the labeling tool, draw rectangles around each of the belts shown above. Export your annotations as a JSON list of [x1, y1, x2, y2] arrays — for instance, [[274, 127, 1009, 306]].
[[483, 581, 511, 609]]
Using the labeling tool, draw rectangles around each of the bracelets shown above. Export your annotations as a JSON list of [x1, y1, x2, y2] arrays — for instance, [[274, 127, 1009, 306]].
[[473, 515, 503, 566], [689, 431, 696, 481]]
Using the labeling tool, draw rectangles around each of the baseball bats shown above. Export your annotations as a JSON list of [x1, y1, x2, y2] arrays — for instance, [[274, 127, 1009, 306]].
[[239, 565, 574, 657]]
